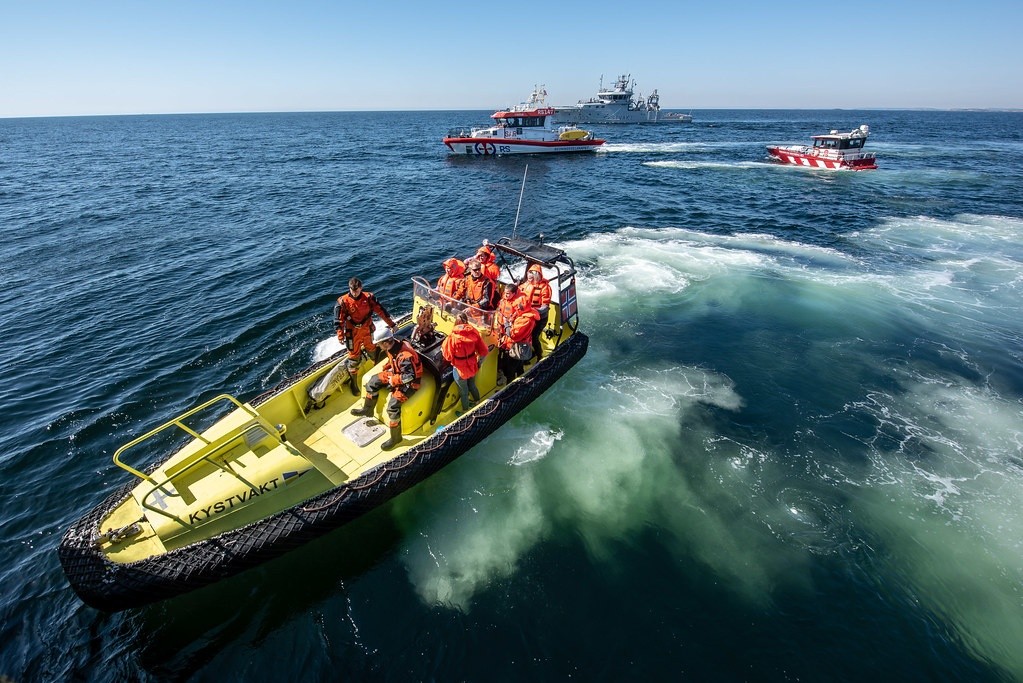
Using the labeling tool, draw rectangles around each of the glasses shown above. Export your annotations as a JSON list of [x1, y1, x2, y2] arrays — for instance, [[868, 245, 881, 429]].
[[472, 267, 481, 271]]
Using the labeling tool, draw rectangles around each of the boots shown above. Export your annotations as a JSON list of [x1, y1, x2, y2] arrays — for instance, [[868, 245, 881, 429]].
[[349, 374, 361, 396], [351, 395, 378, 416], [381, 421, 403, 451]]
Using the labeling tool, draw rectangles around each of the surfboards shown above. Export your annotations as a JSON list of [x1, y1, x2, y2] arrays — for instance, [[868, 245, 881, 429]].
[[559, 131, 589, 139]]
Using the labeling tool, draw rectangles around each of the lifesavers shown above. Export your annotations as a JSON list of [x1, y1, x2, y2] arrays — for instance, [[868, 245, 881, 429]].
[[811, 148, 820, 157]]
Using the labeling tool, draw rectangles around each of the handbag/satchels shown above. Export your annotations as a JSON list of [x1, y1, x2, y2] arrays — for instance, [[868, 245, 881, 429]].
[[509, 342, 531, 360]]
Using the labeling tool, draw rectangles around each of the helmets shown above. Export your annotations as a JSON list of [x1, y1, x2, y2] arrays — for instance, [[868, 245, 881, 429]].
[[371, 328, 393, 344]]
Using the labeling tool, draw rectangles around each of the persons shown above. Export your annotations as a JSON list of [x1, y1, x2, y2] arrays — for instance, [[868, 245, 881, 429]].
[[351, 328, 422, 451], [433, 246, 500, 324], [495, 264, 552, 386], [333, 278, 399, 398], [442, 312, 488, 413]]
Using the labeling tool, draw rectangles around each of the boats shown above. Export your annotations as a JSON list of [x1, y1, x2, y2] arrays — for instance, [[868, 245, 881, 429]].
[[443, 81, 608, 155], [55, 166, 589, 613], [767, 123, 880, 170], [551, 74, 694, 125]]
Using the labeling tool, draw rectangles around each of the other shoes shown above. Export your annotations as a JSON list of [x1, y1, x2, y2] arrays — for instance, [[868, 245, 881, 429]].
[[455, 411, 464, 417], [468, 400, 480, 406]]
